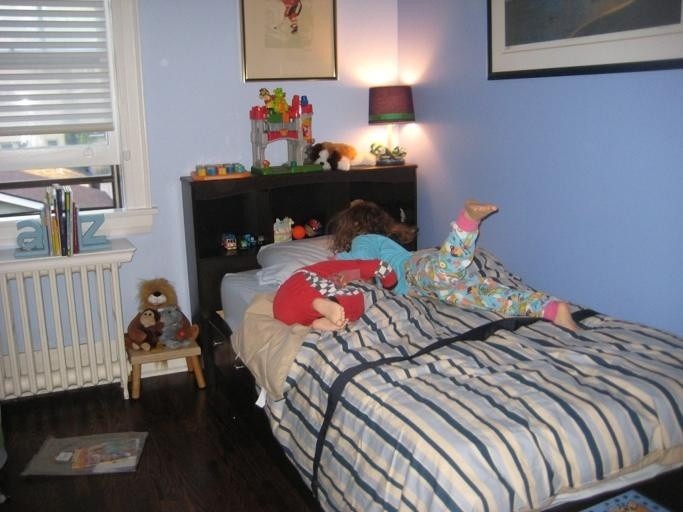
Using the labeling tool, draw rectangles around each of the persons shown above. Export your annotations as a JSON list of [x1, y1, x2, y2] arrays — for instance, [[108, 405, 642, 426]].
[[272, 259, 396, 332], [322, 199, 583, 331]]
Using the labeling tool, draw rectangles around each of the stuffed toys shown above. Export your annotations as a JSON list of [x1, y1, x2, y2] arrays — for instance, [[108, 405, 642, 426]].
[[137, 279, 190, 348], [312, 142, 355, 172], [125, 309, 163, 350]]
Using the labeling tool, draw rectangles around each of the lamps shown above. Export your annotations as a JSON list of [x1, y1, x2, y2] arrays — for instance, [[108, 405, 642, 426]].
[[368, 85, 415, 165]]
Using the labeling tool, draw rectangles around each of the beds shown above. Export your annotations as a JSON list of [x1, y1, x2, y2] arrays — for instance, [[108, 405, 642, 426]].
[[221, 247, 683, 512]]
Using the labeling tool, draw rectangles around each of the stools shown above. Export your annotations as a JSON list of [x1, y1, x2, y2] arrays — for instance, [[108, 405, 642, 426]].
[[128, 340, 206, 399]]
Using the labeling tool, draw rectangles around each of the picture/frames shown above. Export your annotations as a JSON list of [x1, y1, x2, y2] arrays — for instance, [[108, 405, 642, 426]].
[[240, 0, 338, 83], [487, 0, 683, 80]]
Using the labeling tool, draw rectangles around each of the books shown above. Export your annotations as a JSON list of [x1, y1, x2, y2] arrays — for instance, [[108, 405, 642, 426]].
[[42, 183, 78, 257]]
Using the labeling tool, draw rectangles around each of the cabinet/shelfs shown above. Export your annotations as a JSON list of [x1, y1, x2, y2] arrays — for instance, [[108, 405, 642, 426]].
[[180, 163, 418, 388]]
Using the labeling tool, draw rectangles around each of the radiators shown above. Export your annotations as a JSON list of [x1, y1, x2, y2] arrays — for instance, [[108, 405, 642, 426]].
[[0, 238, 138, 400]]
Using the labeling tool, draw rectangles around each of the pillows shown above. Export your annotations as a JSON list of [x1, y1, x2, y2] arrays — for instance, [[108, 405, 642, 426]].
[[256, 234, 340, 284]]
